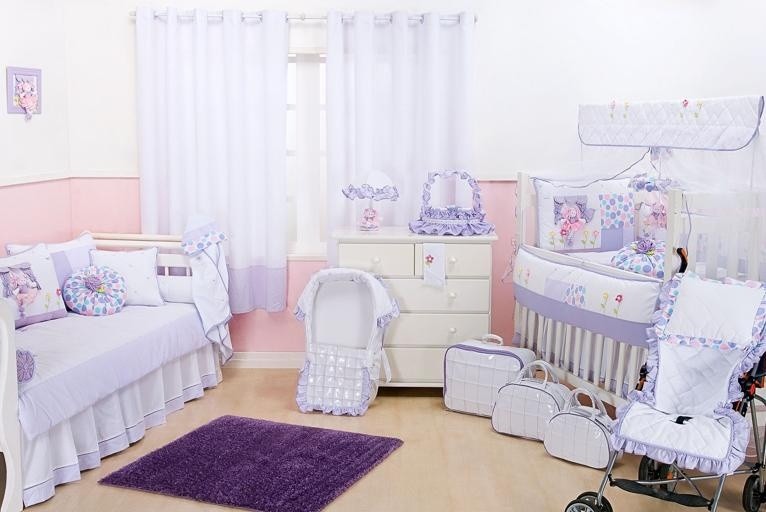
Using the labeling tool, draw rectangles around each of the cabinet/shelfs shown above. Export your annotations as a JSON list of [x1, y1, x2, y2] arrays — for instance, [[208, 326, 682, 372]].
[[328, 236, 498, 388]]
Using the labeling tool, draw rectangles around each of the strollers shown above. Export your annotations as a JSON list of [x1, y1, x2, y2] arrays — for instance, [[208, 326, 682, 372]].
[[566, 272, 766, 512]]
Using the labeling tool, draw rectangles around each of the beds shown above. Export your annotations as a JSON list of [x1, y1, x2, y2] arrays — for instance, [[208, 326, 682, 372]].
[[1, 232, 232, 508], [513, 172, 766, 410]]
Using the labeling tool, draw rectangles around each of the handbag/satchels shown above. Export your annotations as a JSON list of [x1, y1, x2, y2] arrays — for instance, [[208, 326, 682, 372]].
[[543, 388, 623, 471], [442, 332, 536, 418], [490, 360, 581, 443]]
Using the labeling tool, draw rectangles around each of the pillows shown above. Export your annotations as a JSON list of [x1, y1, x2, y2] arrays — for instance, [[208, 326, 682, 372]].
[[1, 242, 67, 329], [62, 265, 127, 315], [7, 230, 99, 292], [609, 242, 681, 280], [88, 249, 163, 306], [532, 177, 636, 253], [16, 349, 35, 386]]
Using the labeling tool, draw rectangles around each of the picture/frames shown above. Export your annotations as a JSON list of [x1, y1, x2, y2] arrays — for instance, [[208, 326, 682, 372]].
[[5, 67, 42, 115]]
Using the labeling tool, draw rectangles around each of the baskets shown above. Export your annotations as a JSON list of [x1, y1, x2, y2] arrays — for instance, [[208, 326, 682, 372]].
[[408, 168, 496, 235]]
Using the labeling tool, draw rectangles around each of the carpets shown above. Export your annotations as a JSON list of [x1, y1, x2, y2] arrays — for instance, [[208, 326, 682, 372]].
[[97, 415, 404, 512]]
